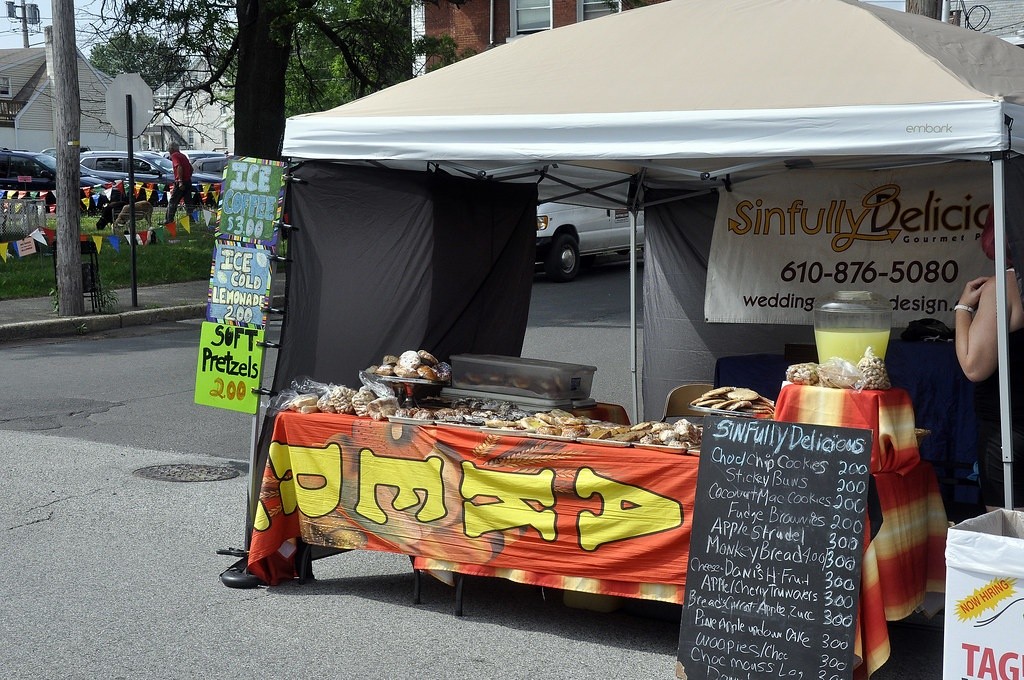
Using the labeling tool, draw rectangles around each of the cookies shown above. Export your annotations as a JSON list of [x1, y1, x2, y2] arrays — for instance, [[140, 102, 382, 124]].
[[690, 386, 775, 414]]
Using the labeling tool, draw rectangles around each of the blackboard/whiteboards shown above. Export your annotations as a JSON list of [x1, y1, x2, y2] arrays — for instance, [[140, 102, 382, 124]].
[[675, 415, 876, 680]]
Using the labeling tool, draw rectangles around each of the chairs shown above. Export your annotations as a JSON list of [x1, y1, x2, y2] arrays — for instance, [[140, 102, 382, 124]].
[[52, 240, 104, 313], [655, 383, 716, 426]]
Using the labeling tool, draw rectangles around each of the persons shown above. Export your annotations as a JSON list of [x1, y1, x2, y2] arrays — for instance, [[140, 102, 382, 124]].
[[158, 141, 195, 224], [954, 204, 1024, 512]]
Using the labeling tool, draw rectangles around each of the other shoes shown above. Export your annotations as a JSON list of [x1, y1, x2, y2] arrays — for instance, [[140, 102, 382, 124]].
[[158, 219, 172, 225], [190, 220, 195, 223]]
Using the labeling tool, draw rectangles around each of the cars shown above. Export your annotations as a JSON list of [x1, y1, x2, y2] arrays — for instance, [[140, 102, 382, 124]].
[[535, 201, 647, 284], [40, 147, 60, 160], [0, 147, 117, 203], [156, 150, 229, 175]]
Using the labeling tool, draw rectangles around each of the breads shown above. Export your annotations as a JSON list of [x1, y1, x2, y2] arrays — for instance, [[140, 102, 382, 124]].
[[464, 370, 567, 391], [288, 394, 319, 413], [392, 396, 702, 452], [366, 349, 452, 381]]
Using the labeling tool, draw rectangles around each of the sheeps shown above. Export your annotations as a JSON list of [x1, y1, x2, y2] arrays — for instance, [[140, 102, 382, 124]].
[[96, 200, 153, 235]]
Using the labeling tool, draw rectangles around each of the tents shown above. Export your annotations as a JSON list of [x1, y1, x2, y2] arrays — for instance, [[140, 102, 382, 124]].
[[243, 0, 1024, 600]]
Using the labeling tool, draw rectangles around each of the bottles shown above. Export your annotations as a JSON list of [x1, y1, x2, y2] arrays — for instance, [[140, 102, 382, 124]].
[[813, 290, 893, 365]]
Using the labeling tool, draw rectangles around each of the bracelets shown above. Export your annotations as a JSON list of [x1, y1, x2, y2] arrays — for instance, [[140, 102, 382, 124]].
[[954, 305, 974, 313]]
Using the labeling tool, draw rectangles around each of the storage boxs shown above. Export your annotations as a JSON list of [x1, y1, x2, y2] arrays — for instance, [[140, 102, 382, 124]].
[[448, 354, 598, 400]]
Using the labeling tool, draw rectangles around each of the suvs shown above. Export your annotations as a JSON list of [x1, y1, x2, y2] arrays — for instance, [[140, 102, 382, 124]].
[[79, 149, 225, 207]]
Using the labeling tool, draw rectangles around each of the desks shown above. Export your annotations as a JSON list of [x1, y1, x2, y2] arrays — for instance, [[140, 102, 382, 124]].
[[249, 411, 952, 680]]
